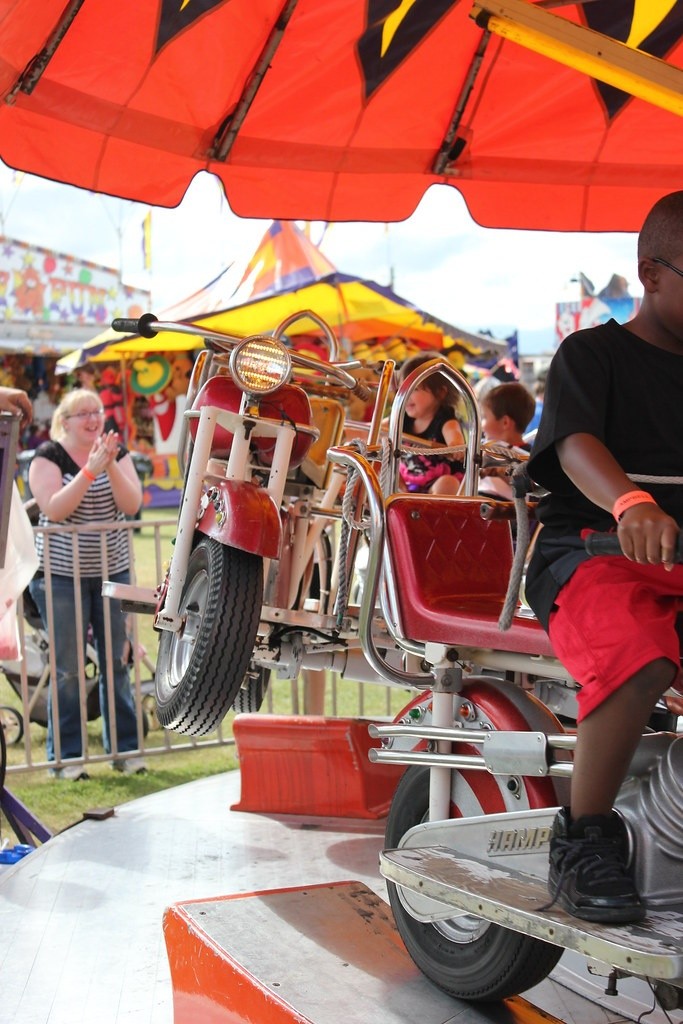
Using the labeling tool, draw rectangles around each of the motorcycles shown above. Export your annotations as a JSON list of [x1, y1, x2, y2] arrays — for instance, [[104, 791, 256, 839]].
[[325, 425, 541, 636], [112, 306, 476, 737], [230, 485, 683, 1024]]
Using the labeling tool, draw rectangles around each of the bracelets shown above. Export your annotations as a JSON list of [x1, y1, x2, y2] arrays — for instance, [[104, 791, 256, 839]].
[[82, 467, 96, 482], [613, 490, 658, 524]]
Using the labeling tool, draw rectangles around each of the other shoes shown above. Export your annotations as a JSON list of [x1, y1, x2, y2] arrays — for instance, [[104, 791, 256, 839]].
[[45, 763, 90, 782], [108, 756, 150, 774]]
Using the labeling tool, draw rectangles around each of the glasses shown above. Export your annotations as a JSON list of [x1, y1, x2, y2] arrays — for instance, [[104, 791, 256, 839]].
[[64, 408, 105, 420]]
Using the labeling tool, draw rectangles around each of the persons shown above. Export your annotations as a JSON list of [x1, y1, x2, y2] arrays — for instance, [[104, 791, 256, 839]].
[[0, 386, 148, 778], [359, 356, 469, 494], [525, 369, 549, 444], [481, 383, 535, 534], [526, 190, 683, 923]]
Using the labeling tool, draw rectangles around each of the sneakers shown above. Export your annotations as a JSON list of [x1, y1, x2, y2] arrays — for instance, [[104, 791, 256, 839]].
[[533, 805, 648, 924]]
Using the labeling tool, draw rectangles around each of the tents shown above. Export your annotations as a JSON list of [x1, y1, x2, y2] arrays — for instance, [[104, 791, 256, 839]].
[[49, 218, 509, 445]]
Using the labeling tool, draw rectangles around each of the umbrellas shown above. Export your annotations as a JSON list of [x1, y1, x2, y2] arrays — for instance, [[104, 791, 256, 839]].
[[3, 0, 683, 233]]
